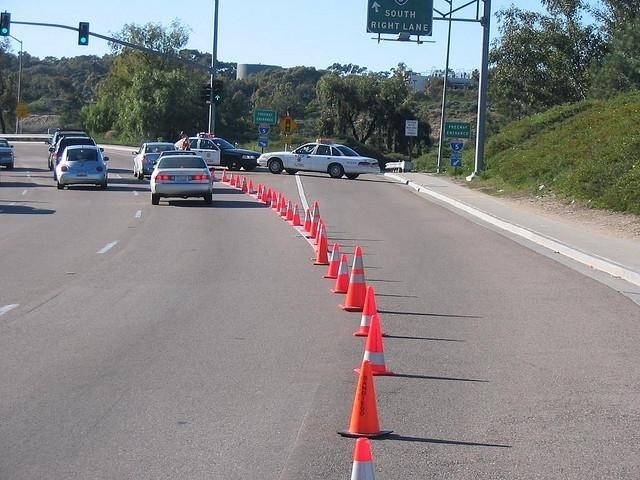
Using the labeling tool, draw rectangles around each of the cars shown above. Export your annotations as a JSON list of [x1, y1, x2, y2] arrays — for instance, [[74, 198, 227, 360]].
[[45, 127, 111, 189], [0, 138, 15, 168], [133, 131, 380, 203]]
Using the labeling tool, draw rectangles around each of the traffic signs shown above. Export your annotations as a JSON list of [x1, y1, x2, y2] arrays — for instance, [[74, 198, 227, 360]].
[[368, 0, 433, 37], [255, 110, 275, 123], [444, 122, 469, 138]]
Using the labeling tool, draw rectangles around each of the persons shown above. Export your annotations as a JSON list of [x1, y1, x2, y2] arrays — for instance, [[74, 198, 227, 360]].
[[178, 131, 192, 151]]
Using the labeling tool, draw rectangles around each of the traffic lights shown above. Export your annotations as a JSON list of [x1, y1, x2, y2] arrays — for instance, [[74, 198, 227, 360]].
[[1, 13, 10, 34], [80, 24, 88, 44], [201, 84, 209, 102], [285, 119, 290, 132], [214, 82, 222, 101]]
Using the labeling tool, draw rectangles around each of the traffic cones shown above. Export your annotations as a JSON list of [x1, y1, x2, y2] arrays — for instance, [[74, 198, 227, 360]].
[[337, 360, 395, 441], [352, 285, 387, 337], [351, 314, 396, 378], [351, 436, 376, 480], [337, 247, 367, 311], [216, 168, 351, 295]]
